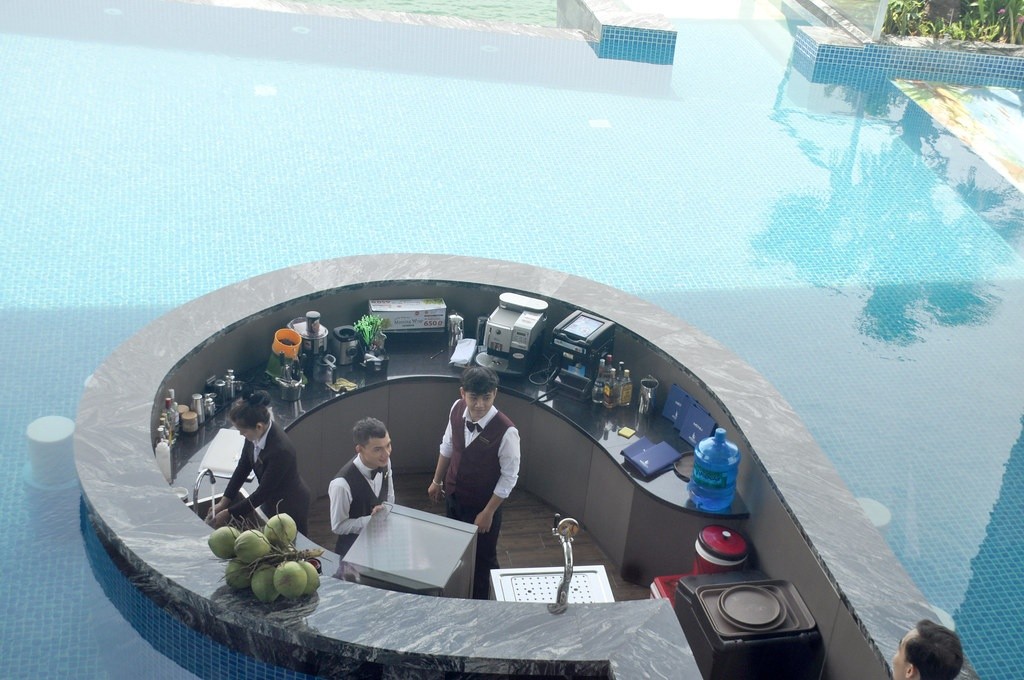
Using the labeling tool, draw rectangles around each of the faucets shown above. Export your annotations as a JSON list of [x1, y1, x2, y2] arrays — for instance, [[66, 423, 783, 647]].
[[547, 513, 580, 615], [193, 467, 217, 516]]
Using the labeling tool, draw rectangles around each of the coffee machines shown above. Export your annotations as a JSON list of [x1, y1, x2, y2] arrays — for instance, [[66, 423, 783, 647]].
[[286, 310, 337, 385]]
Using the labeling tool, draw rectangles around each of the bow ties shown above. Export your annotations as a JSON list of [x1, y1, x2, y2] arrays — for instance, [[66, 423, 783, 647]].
[[371, 465, 388, 480], [466, 421, 483, 432]]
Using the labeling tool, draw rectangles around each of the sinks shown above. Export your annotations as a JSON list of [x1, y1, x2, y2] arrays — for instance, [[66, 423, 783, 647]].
[[490, 565, 616, 603], [185, 487, 270, 534]]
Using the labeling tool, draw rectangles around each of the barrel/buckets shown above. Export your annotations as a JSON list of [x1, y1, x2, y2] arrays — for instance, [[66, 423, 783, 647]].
[[695, 524, 748, 573]]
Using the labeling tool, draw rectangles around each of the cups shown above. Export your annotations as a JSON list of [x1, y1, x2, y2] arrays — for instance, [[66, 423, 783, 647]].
[[446, 309, 464, 346], [637, 375, 659, 415]]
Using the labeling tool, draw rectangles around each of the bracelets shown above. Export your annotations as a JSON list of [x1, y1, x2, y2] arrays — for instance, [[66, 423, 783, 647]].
[[433, 479, 443, 486]]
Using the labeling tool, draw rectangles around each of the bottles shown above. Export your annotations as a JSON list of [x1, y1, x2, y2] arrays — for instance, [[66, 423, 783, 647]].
[[155, 370, 235, 484], [689, 427, 741, 511], [592, 354, 632, 407]]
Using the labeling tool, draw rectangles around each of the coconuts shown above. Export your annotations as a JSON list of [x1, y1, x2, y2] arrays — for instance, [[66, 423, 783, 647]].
[[208, 513, 320, 602]]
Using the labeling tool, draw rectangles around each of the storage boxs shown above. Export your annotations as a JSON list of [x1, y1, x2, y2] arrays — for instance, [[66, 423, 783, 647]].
[[369, 298, 446, 330]]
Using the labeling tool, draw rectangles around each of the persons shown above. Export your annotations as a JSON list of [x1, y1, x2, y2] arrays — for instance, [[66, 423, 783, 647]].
[[205, 390, 311, 537], [328, 417, 396, 555], [891, 620, 963, 680], [428, 366, 521, 600]]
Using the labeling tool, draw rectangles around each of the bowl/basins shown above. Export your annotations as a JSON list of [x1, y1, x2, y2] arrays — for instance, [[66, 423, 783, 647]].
[[278, 380, 302, 402]]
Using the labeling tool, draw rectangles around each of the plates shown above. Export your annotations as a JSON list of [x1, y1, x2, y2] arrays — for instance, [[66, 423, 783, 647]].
[[717, 587, 786, 631], [721, 586, 780, 624], [699, 585, 800, 635]]
